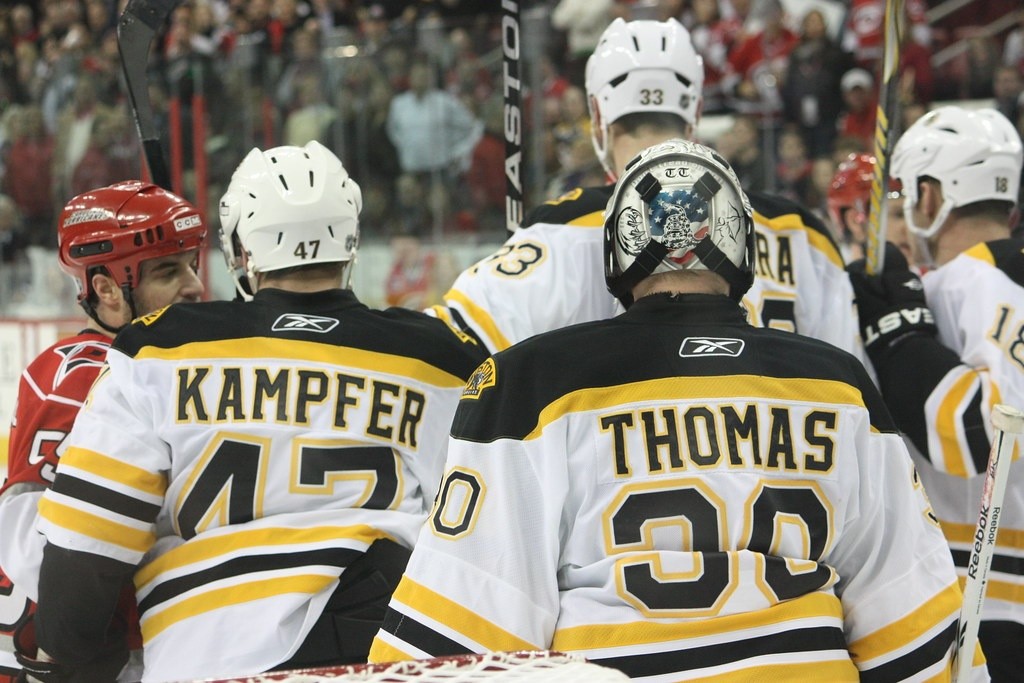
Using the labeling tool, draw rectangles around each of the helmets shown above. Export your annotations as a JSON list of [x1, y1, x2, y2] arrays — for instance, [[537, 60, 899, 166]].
[[57, 179, 205, 304], [585, 17, 705, 126], [890, 106, 1023, 208], [827, 153, 902, 235], [603, 138, 752, 278], [217, 140, 363, 274]]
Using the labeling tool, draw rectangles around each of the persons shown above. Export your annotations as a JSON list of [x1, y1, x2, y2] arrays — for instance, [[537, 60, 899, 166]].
[[0, 179, 208, 683], [368, 138, 993, 683], [0, 0, 1024, 245], [424, 16, 883, 397], [844, 107, 1023, 683], [27, 140, 493, 683], [827, 149, 914, 271]]
